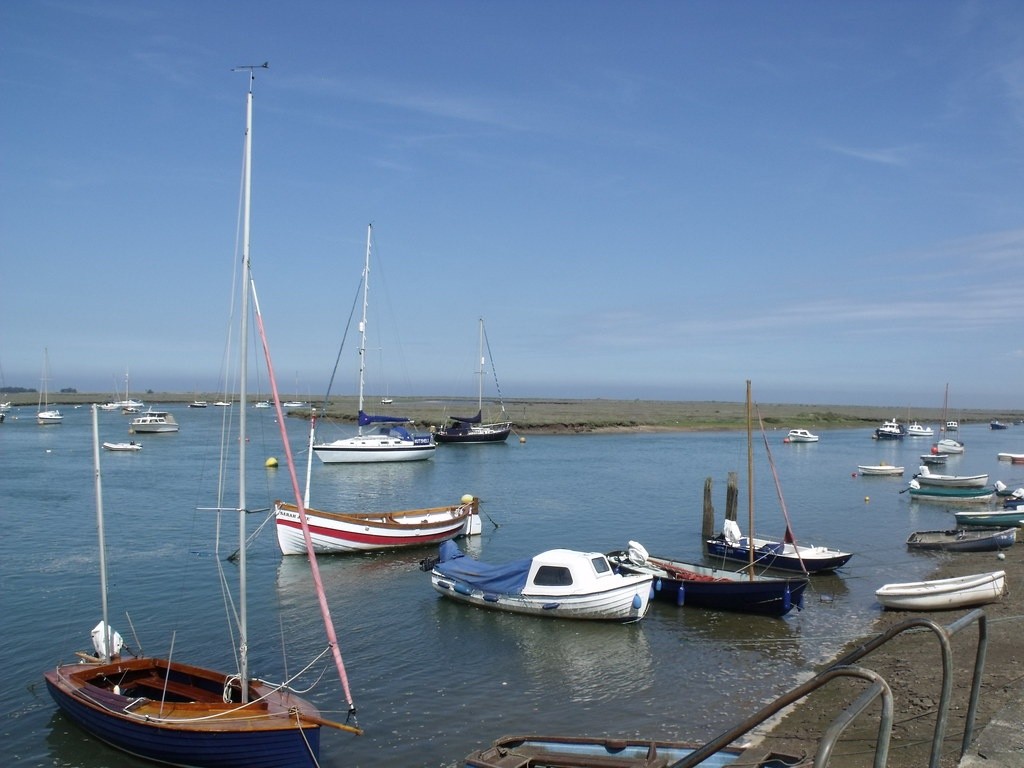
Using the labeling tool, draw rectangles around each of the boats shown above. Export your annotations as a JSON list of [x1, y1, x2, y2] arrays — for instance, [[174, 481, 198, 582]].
[[0, 401, 11, 412], [255, 399, 307, 408], [416, 539, 658, 625], [1013, 419, 1024, 425], [997, 453, 1024, 462], [876, 418, 907, 439], [954, 509, 1024, 527], [947, 421, 958, 431], [918, 453, 949, 464], [113, 366, 144, 415], [857, 460, 905, 477], [911, 464, 990, 487], [128, 410, 180, 432], [102, 441, 143, 451], [784, 427, 820, 444], [905, 525, 1018, 551], [195, 401, 207, 405], [907, 422, 935, 436], [1011, 455, 1024, 465], [702, 520, 855, 574], [97, 403, 120, 410], [463, 734, 813, 768], [989, 420, 1008, 429], [0, 412, 6, 423], [993, 480, 1024, 511], [898, 478, 998, 502], [188, 403, 209, 408], [213, 401, 231, 406], [875, 570, 1007, 609], [269, 416, 485, 555]]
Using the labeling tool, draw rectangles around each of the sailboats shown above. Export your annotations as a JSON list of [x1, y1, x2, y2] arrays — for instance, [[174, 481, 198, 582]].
[[430, 317, 515, 444], [35, 346, 64, 425], [602, 375, 812, 617], [931, 382, 965, 454], [41, 67, 364, 767], [312, 224, 439, 466], [380, 381, 393, 404]]
